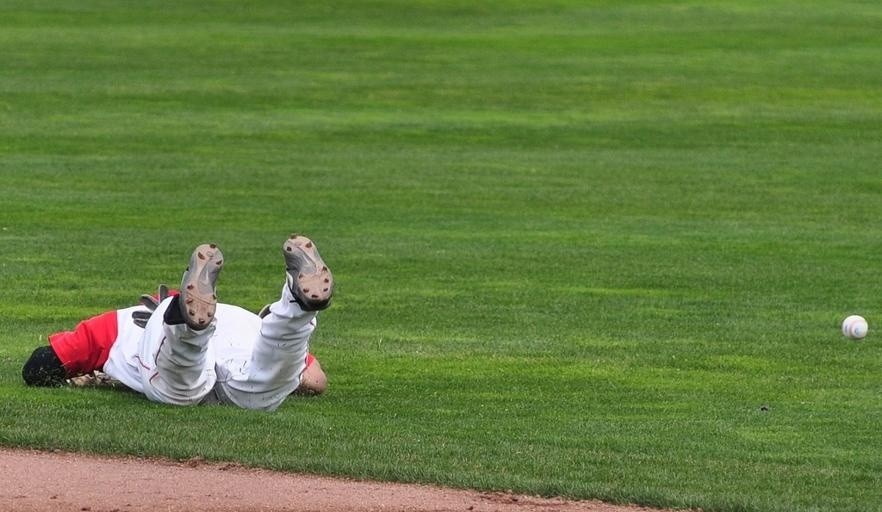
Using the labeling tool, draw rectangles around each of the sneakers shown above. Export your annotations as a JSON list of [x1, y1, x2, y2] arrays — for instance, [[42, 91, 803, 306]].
[[282, 235, 334, 312], [164, 243, 224, 332]]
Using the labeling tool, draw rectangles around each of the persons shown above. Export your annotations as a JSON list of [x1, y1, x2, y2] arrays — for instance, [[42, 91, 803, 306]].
[[22, 232, 333, 412]]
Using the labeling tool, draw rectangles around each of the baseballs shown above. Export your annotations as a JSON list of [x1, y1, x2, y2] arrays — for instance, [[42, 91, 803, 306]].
[[843, 315, 869, 340]]
